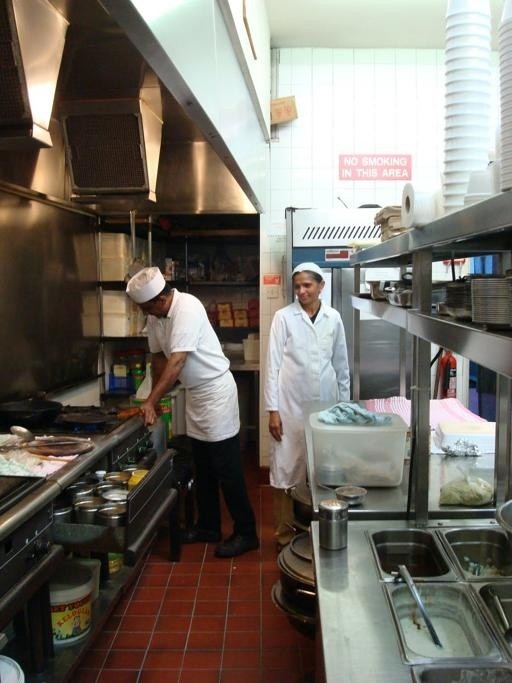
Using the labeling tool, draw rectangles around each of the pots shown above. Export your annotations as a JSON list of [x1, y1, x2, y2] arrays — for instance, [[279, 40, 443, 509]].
[[271, 476, 320, 640], [1, 399, 93, 429], [367, 272, 445, 307]]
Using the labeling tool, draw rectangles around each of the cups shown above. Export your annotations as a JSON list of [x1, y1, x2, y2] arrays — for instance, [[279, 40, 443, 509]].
[[49, 467, 154, 530]]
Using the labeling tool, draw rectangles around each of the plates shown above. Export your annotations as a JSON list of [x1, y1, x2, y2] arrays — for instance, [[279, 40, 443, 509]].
[[444, 278, 512, 327]]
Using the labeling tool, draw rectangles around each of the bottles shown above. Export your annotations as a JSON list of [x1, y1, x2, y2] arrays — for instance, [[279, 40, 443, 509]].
[[317, 499, 349, 550], [132, 363, 145, 393]]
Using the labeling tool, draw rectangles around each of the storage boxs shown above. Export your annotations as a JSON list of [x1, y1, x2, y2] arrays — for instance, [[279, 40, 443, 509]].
[[310, 411, 408, 490], [96, 231, 152, 338]]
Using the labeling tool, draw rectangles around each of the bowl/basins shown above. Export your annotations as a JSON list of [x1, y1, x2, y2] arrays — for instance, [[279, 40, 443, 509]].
[[441, 0, 511, 217], [335, 485, 368, 506]]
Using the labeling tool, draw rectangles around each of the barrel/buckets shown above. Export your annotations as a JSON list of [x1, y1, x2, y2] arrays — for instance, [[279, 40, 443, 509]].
[[50, 574, 93, 644]]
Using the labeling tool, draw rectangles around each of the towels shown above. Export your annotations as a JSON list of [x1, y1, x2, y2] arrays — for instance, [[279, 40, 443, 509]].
[[319, 397, 388, 425]]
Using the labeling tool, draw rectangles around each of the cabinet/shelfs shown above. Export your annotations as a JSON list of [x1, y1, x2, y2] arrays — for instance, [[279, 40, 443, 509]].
[[347, 189, 512, 379], [167, 237, 259, 343]]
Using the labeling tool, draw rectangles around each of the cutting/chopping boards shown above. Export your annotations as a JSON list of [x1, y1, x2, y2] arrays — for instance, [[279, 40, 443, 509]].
[[29, 437, 95, 456]]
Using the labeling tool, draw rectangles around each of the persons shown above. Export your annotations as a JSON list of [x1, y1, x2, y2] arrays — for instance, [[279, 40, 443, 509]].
[[264, 261, 352, 552], [125, 264, 261, 559]]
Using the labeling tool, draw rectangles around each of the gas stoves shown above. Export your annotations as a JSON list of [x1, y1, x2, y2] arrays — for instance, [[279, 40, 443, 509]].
[[0, 390, 143, 435]]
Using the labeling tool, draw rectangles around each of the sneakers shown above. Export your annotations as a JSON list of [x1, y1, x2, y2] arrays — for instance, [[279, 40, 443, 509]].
[[180, 526, 221, 543], [215, 532, 259, 558]]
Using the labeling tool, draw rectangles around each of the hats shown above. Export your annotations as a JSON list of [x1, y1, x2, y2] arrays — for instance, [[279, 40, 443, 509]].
[[125, 265, 166, 304], [292, 261, 324, 278]]
